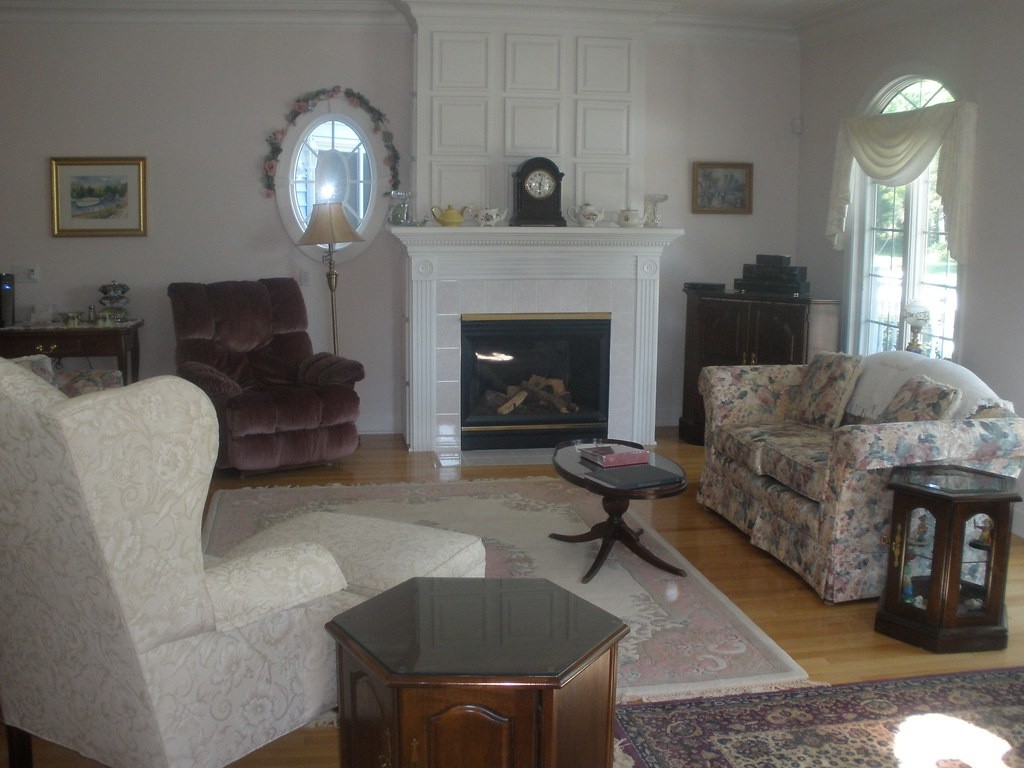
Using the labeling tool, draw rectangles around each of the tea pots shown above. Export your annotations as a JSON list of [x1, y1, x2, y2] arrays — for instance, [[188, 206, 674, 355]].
[[567, 205, 606, 228], [469, 206, 509, 227], [431, 204, 468, 226], [611, 209, 650, 228]]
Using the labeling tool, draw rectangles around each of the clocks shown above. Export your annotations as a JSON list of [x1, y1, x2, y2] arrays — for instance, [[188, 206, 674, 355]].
[[508, 157, 567, 227]]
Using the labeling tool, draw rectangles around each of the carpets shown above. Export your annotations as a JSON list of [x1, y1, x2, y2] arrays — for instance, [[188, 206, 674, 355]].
[[201, 475, 810, 704], [616, 664, 1024, 768]]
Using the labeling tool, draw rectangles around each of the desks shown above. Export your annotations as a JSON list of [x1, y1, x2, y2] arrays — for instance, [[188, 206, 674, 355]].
[[324, 577, 631, 768], [0, 317, 145, 387], [873, 464, 1024, 652], [548, 438, 688, 583]]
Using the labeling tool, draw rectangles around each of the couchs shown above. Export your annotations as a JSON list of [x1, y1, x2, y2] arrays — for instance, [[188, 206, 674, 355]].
[[695, 350, 1024, 607]]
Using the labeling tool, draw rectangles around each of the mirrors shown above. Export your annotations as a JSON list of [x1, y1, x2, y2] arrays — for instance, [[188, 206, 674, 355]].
[[260, 85, 401, 266]]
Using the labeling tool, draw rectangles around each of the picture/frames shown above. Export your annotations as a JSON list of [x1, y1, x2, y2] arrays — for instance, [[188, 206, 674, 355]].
[[692, 161, 753, 214], [49, 155, 147, 236]]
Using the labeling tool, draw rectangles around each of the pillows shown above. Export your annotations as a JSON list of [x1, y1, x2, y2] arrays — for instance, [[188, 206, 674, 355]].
[[783, 351, 864, 432], [874, 374, 961, 423]]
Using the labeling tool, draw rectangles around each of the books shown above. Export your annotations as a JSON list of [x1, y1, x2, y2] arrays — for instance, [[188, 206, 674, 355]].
[[578, 445, 650, 468], [584, 463, 683, 489]]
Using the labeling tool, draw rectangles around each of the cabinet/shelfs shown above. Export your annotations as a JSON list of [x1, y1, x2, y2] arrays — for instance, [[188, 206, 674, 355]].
[[678, 282, 840, 446]]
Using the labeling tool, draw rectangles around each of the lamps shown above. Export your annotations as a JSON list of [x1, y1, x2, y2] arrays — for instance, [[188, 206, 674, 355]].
[[292, 202, 368, 356], [902, 298, 929, 355]]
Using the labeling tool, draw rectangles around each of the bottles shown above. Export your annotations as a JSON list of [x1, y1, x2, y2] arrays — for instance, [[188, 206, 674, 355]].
[[88, 304, 96, 324]]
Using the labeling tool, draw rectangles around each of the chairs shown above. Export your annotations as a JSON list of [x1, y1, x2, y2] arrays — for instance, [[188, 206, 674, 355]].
[[167, 277, 365, 478], [0, 356, 486, 768]]
